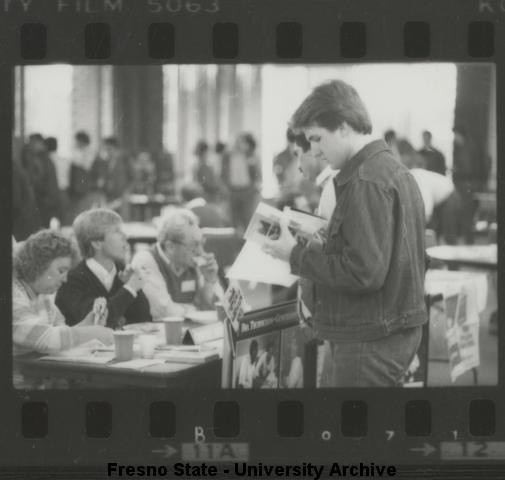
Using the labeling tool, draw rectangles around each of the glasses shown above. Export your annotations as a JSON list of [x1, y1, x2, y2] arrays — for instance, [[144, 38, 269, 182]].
[[172, 238, 207, 251]]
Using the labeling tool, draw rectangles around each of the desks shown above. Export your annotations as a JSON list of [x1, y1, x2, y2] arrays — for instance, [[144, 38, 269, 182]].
[[432, 243, 500, 336], [33, 321, 222, 389], [421, 268, 488, 387]]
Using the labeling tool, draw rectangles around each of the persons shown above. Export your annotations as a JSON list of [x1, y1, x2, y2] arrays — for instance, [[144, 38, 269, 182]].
[[258, 79, 428, 387], [55, 207, 153, 327], [282, 124, 339, 386], [122, 208, 219, 316], [271, 148, 324, 215], [11, 131, 263, 242], [12, 230, 115, 354], [238, 334, 306, 390], [384, 122, 493, 190], [410, 166, 473, 246]]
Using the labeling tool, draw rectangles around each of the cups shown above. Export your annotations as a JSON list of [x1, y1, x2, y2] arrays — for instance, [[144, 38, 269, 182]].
[[113, 331, 133, 361], [164, 317, 184, 344]]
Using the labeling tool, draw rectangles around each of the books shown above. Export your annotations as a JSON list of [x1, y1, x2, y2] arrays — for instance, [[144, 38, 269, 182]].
[[242, 201, 319, 262], [154, 343, 218, 364]]
